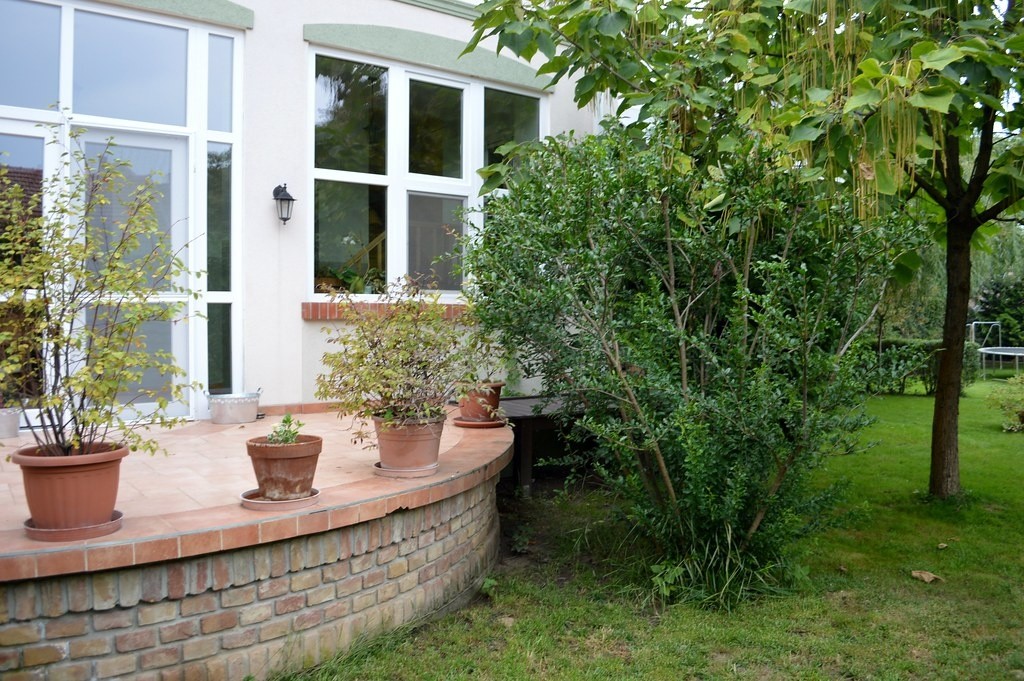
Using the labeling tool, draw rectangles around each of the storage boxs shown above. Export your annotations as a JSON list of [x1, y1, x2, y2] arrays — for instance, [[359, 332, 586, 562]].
[[203, 386, 264, 424]]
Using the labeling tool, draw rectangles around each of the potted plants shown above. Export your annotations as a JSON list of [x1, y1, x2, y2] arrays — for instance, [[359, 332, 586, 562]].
[[312, 272, 486, 479], [449, 278, 508, 428], [0, 100, 213, 542], [239, 413, 323, 511]]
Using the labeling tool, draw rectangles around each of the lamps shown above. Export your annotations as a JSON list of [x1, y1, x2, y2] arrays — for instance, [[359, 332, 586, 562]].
[[272, 182, 297, 226]]
